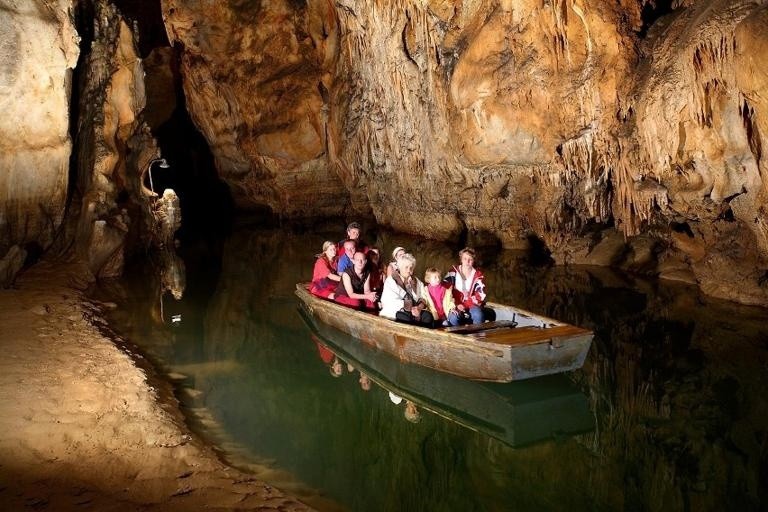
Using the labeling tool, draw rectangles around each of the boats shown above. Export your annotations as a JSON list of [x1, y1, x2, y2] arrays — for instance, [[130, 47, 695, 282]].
[[294, 282, 597, 383]]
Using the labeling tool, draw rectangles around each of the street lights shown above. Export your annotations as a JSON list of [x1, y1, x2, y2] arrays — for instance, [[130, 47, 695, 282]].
[[160, 270, 182, 326]]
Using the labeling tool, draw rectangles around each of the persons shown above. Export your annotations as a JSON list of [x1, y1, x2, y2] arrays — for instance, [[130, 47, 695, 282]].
[[386, 246, 406, 278], [333, 249, 379, 309], [424, 267, 457, 321], [336, 238, 358, 276], [364, 246, 386, 284], [440, 246, 489, 327], [311, 337, 425, 425], [310, 240, 341, 300], [336, 221, 368, 258], [377, 253, 433, 329]]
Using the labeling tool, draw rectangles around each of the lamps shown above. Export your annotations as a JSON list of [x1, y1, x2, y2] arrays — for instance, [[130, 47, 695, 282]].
[[161, 290, 183, 327]]
